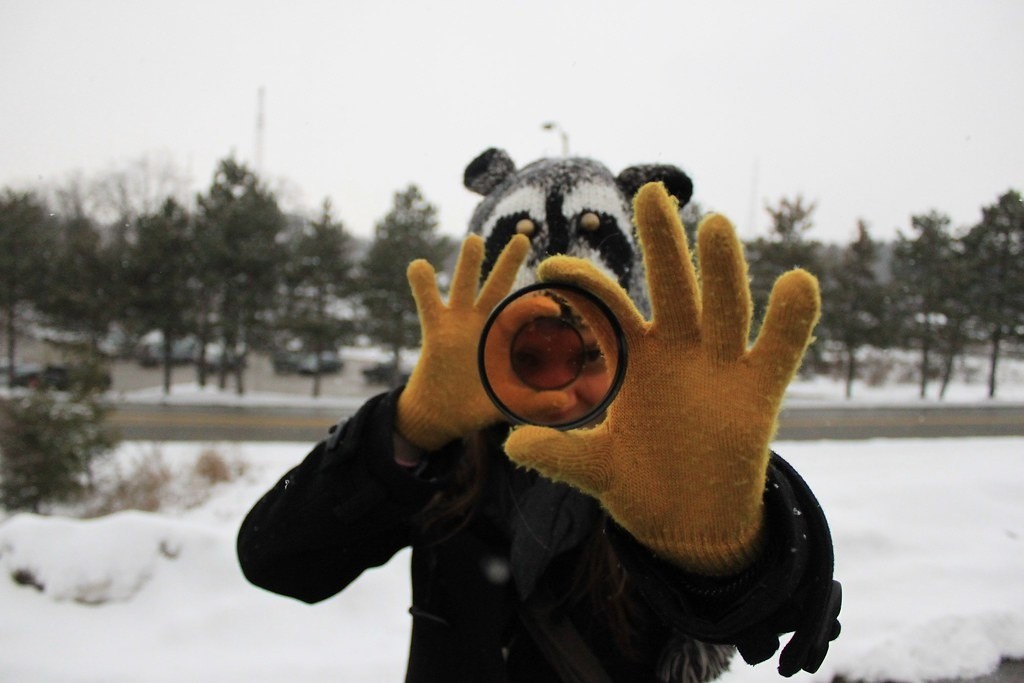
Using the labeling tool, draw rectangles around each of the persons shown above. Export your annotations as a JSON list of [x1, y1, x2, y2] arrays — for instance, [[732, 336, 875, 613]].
[[237, 145, 836, 683]]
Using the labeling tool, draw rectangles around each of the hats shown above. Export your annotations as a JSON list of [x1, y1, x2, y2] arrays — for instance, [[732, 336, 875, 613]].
[[465, 147, 693, 301]]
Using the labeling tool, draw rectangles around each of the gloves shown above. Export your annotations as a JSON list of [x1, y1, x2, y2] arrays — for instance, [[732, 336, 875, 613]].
[[396, 233, 576, 448], [502, 182, 822, 574]]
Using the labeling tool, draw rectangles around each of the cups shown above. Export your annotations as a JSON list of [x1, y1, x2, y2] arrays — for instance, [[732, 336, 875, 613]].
[[476, 281, 628, 433]]
